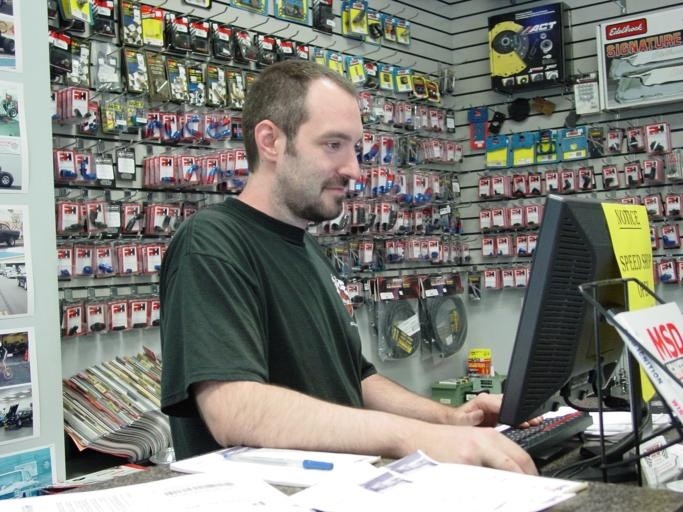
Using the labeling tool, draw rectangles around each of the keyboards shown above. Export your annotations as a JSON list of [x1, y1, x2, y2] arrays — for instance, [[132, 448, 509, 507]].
[[499, 410, 594, 455]]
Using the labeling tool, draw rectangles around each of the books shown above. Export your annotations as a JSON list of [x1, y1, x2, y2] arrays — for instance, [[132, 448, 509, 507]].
[[63, 344, 173, 462]]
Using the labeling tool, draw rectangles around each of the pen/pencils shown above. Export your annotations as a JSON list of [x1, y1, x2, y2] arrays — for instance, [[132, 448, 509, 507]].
[[224, 454, 333, 470]]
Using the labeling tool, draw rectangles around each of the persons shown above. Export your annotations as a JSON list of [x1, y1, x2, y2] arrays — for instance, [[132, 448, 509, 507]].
[[159, 57, 544, 479]]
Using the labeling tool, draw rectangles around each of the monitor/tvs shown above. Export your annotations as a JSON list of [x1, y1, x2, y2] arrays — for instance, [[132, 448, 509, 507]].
[[497, 194, 659, 481]]
